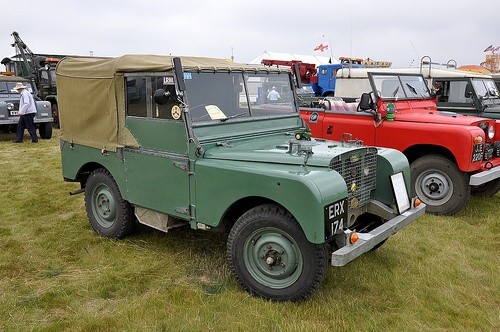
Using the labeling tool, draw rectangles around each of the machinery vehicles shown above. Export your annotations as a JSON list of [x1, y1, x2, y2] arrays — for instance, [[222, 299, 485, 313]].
[[263, 57, 318, 88], [9, 31, 60, 128]]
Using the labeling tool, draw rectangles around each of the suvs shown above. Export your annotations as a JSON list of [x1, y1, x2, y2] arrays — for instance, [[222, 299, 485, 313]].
[[54, 53, 427, 300], [1, 71, 54, 141], [411, 68, 500, 121]]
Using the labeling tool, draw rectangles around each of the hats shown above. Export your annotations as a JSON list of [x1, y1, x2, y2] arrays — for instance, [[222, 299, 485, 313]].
[[13, 82, 27, 90]]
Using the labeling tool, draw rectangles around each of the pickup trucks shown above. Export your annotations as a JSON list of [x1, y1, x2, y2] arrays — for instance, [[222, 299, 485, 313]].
[[243, 69, 500, 217]]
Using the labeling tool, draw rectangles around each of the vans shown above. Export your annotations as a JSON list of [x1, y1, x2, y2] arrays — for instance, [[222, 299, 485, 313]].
[[313, 63, 370, 99]]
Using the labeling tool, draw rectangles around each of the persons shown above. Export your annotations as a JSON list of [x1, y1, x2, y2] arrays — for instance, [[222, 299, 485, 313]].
[[13, 82, 39, 145], [265, 86, 283, 104], [306, 69, 310, 81]]
